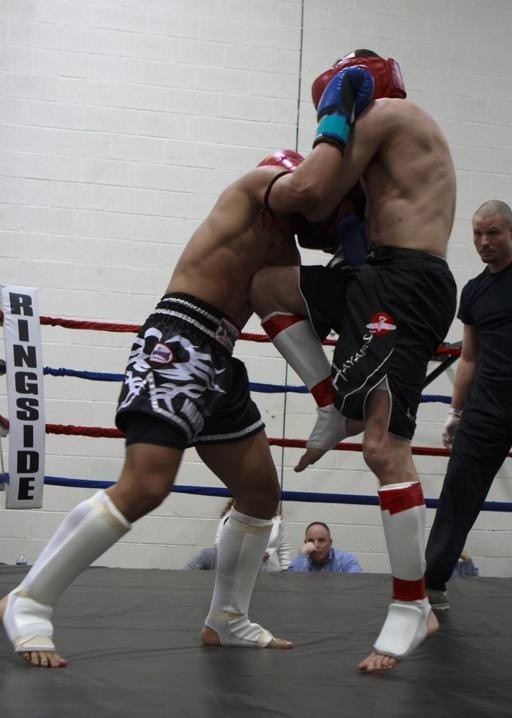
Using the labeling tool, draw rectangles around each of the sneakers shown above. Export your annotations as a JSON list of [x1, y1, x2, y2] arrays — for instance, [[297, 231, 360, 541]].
[[424, 587, 451, 613]]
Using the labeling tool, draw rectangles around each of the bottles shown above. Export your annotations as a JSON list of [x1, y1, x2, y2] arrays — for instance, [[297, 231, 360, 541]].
[[16, 554, 28, 565]]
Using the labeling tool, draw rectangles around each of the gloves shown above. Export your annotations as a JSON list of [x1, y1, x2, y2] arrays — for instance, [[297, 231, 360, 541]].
[[440, 407, 463, 450], [256, 148, 304, 173], [312, 64, 375, 157]]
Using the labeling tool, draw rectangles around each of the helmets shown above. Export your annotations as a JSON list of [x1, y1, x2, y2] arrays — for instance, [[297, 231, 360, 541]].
[[310, 54, 407, 106]]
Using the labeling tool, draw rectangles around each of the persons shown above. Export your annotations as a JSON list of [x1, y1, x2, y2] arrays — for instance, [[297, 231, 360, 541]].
[[244, 48, 458, 681], [416, 197, 512, 612], [2, 63, 380, 673], [289, 522, 363, 575], [183, 516, 232, 573], [448, 549, 479, 578], [217, 492, 292, 575]]
